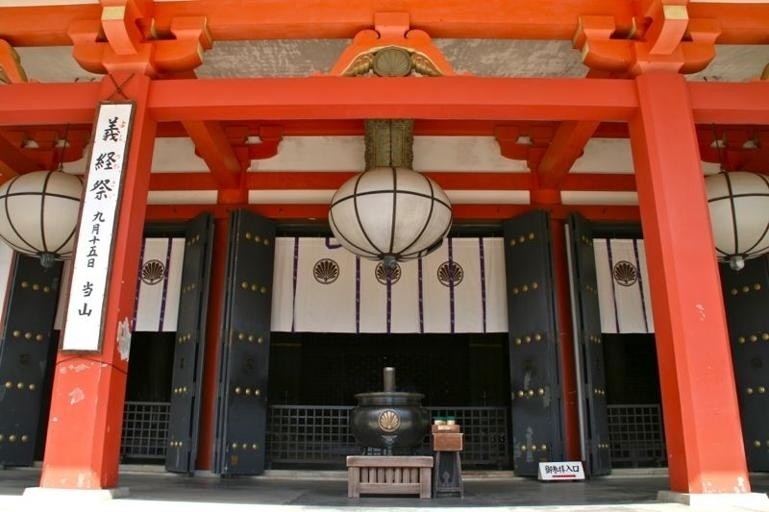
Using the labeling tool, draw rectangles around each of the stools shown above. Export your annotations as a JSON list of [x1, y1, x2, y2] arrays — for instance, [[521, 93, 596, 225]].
[[431, 451, 467, 500]]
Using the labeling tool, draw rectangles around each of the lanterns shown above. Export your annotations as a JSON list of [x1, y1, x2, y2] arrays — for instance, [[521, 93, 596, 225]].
[[327, 161, 454, 271], [703, 170, 769, 273], [0, 165, 87, 269]]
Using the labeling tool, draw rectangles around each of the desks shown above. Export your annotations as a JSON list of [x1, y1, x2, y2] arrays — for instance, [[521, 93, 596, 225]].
[[345, 455, 434, 500]]
